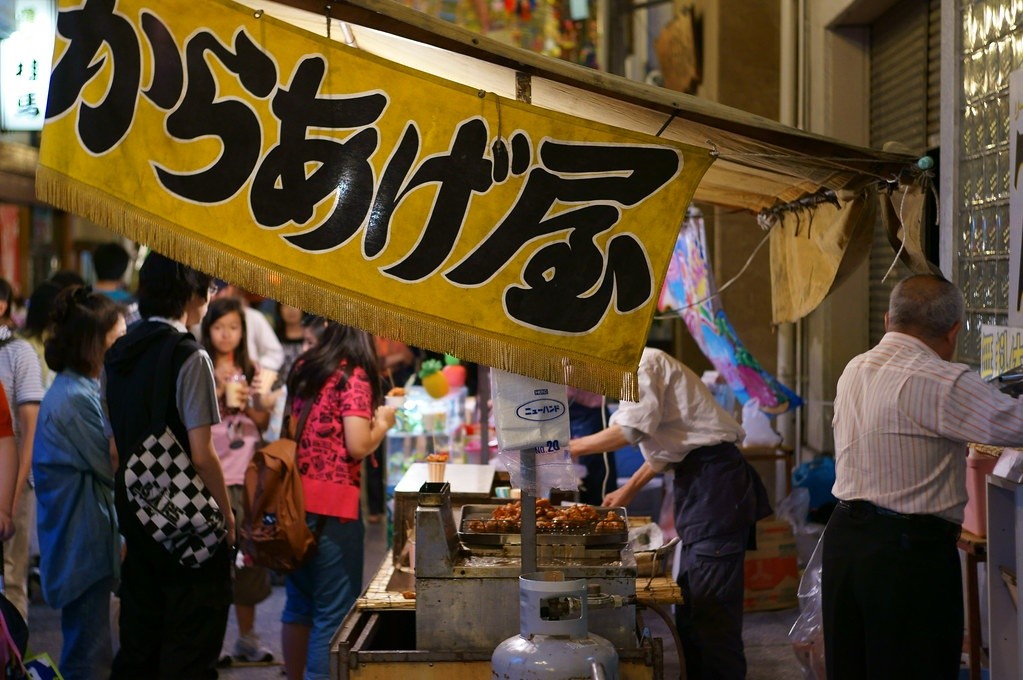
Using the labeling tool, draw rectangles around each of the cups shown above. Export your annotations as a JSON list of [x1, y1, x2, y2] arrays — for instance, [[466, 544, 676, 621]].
[[495, 487, 511, 499], [394, 408, 417, 431], [423, 410, 445, 432], [226, 375, 246, 407], [429, 462, 445, 482], [385, 395, 406, 409], [510, 489, 521, 498]]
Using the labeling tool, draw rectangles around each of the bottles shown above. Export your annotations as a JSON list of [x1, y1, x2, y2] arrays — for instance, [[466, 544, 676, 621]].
[[433, 423, 482, 465]]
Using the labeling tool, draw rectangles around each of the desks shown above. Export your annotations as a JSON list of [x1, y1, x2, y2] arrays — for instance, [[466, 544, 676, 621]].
[[958, 531, 987, 680]]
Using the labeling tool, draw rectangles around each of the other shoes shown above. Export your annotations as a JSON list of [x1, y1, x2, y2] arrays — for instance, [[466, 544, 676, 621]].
[[233, 637, 273, 664], [218, 648, 232, 666]]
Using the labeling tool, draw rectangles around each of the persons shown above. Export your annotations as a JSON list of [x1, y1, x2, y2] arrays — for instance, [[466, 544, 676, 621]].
[[213, 279, 284, 374], [199, 297, 274, 668], [271, 302, 309, 392], [282, 323, 396, 680], [302, 313, 332, 349], [91, 242, 129, 302], [372, 336, 411, 392], [0, 280, 42, 638], [822, 274, 1023, 680], [99, 250, 234, 680], [21, 271, 78, 389], [569, 346, 771, 680], [31, 294, 126, 680]]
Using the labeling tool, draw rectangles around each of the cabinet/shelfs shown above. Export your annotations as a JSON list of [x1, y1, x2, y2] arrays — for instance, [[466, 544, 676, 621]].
[[985, 472, 1023, 680]]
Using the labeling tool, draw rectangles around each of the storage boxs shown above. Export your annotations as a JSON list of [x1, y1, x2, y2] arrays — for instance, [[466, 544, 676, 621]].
[[960, 456, 998, 538], [741, 520, 800, 613], [385, 385, 467, 434]]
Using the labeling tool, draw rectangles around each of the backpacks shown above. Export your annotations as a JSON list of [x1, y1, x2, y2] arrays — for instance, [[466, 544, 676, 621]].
[[241, 356, 343, 566]]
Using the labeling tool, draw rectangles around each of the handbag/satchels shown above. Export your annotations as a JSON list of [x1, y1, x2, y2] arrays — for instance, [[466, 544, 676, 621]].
[[115, 422, 226, 568], [0, 612, 64, 680]]
